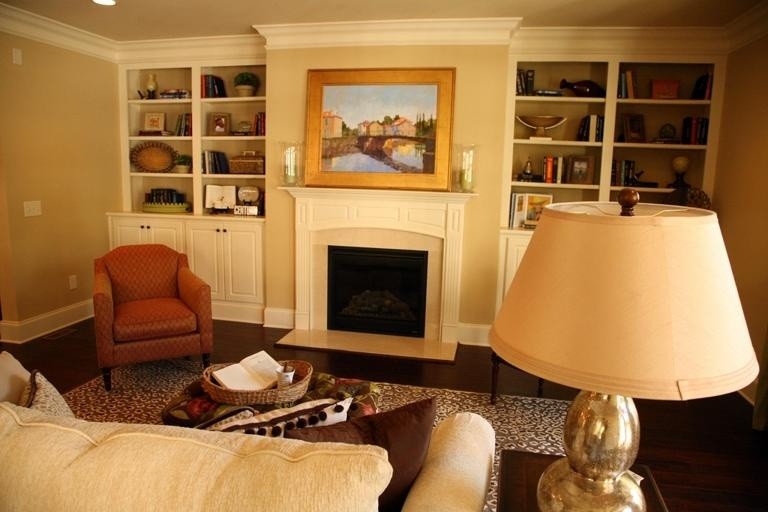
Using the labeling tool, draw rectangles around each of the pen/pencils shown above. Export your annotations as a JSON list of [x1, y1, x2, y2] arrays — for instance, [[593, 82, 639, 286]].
[[284, 361, 287, 372]]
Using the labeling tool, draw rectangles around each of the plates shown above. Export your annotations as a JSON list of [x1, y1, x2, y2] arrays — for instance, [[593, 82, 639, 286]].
[[237, 185, 262, 204], [535, 88, 564, 97]]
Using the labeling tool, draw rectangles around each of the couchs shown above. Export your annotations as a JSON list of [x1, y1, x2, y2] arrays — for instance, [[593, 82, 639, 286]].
[[1, 352, 497, 511], [92, 244, 212, 391]]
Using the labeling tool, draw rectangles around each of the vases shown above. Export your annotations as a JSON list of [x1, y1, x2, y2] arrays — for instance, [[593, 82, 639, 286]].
[[280, 142, 298, 185], [453, 141, 477, 193], [559, 78, 604, 98]]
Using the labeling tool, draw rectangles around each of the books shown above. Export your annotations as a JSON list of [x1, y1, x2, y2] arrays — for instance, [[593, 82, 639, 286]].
[[543, 155, 563, 183], [204, 150, 228, 174], [254, 111, 265, 136], [514, 69, 535, 96], [200, 74, 228, 98], [682, 114, 707, 145], [210, 350, 285, 394], [616, 71, 639, 98], [172, 113, 192, 136], [510, 190, 552, 228], [609, 160, 636, 185], [576, 113, 603, 139], [145, 188, 185, 208]]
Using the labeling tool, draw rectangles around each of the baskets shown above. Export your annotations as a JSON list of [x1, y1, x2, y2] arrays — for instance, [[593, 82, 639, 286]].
[[229, 151, 264, 174], [203, 360, 313, 405]]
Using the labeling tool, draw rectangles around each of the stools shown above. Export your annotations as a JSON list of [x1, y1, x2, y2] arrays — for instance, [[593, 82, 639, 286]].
[[161, 374, 379, 424]]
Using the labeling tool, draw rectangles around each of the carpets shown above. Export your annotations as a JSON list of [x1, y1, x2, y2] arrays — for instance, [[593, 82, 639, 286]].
[[61, 358, 572, 511]]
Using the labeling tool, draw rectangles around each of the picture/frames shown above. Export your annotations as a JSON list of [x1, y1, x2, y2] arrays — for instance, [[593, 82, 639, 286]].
[[206, 110, 232, 138], [141, 112, 166, 130], [303, 66, 458, 192], [622, 110, 647, 143], [565, 155, 596, 184]]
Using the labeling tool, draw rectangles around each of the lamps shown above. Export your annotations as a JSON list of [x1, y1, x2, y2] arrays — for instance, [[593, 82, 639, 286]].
[[665, 155, 691, 188], [488, 186, 761, 512]]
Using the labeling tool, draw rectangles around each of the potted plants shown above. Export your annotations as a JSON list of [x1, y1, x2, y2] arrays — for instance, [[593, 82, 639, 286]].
[[173, 153, 191, 174], [233, 73, 259, 97]]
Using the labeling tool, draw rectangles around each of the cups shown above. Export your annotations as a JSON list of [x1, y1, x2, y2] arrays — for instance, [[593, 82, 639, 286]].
[[449, 142, 476, 194], [274, 366, 294, 389], [279, 142, 306, 188]]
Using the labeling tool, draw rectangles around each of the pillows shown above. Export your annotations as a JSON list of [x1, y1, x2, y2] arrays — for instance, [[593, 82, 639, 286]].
[[208, 392, 359, 439], [284, 396, 439, 511]]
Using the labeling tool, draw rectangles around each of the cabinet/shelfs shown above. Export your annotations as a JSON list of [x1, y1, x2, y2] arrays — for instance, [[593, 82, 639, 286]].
[[499, 53, 728, 230], [107, 212, 185, 255], [496, 446, 668, 512], [184, 213, 267, 323], [118, 58, 266, 215]]
